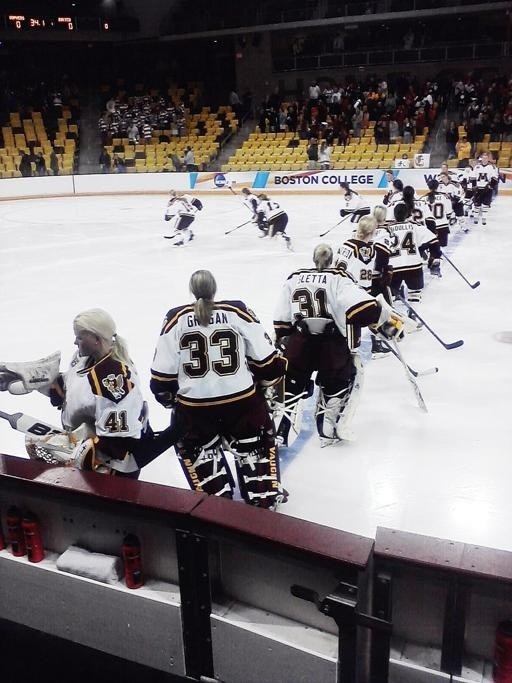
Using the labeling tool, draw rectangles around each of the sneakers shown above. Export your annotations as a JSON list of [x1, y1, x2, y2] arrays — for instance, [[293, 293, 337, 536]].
[[371, 336, 393, 355], [431, 271, 444, 279], [172, 231, 194, 247], [281, 232, 293, 251], [449, 210, 487, 232]]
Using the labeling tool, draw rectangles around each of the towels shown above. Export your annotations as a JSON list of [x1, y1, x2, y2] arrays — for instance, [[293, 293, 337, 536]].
[[56, 544, 124, 585]]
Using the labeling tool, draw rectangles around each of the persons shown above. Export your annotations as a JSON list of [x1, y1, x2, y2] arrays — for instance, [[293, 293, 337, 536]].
[[47, 75, 81, 107], [49, 151, 58, 176], [336, 151, 512, 311], [18, 149, 32, 175], [260, 64, 512, 167], [148, 267, 286, 517], [164, 190, 203, 246], [26, 307, 152, 480], [241, 187, 295, 253], [98, 77, 257, 174], [327, 212, 394, 360], [35, 151, 46, 175], [270, 236, 384, 444]]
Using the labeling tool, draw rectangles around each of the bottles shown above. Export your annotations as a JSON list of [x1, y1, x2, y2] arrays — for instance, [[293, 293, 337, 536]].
[[0, 501, 45, 564], [492, 617, 512, 683], [121, 531, 145, 590]]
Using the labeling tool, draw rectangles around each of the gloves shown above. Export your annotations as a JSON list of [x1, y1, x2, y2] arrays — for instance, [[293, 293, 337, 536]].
[[428, 256, 441, 271]]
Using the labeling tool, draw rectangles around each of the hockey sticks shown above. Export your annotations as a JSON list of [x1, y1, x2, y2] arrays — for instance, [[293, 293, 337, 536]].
[[369, 327, 436, 411], [320, 212, 352, 237], [392, 289, 463, 350], [442, 250, 480, 289], [165, 206, 202, 238], [225, 221, 251, 235]]
